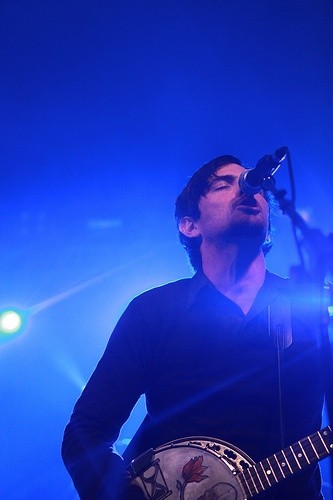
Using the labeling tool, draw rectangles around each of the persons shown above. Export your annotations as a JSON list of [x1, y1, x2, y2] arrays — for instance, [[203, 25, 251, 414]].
[[61, 155, 333, 500]]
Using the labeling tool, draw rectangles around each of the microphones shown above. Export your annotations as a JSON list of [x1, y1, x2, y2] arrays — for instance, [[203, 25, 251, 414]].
[[238, 146, 287, 195]]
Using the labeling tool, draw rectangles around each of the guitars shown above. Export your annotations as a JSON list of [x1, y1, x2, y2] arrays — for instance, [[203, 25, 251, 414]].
[[129, 422, 332, 500]]
